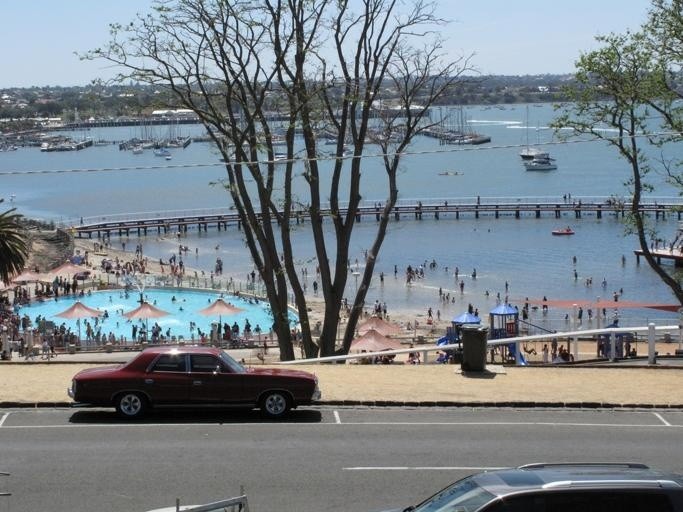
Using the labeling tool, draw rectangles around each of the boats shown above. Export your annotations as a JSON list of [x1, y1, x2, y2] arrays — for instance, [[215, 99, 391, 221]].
[[133, 111, 191, 160], [552, 230, 574, 235], [40, 114, 93, 151], [426, 105, 490, 144], [521, 105, 557, 170]]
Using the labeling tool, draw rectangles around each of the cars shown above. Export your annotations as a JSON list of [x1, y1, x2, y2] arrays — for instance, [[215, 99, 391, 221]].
[[68, 347, 321, 419], [382, 462, 683, 512]]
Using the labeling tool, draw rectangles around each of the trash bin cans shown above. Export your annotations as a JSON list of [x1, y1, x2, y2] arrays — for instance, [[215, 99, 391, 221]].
[[461, 324, 489, 371]]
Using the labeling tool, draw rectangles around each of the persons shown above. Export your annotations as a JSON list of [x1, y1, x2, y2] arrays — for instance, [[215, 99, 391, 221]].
[[562, 193, 571, 202], [608, 198, 616, 207], [0, 244, 637, 364], [567, 226, 571, 231], [444, 201, 448, 206], [419, 202, 422, 211], [650, 238, 659, 252]]
